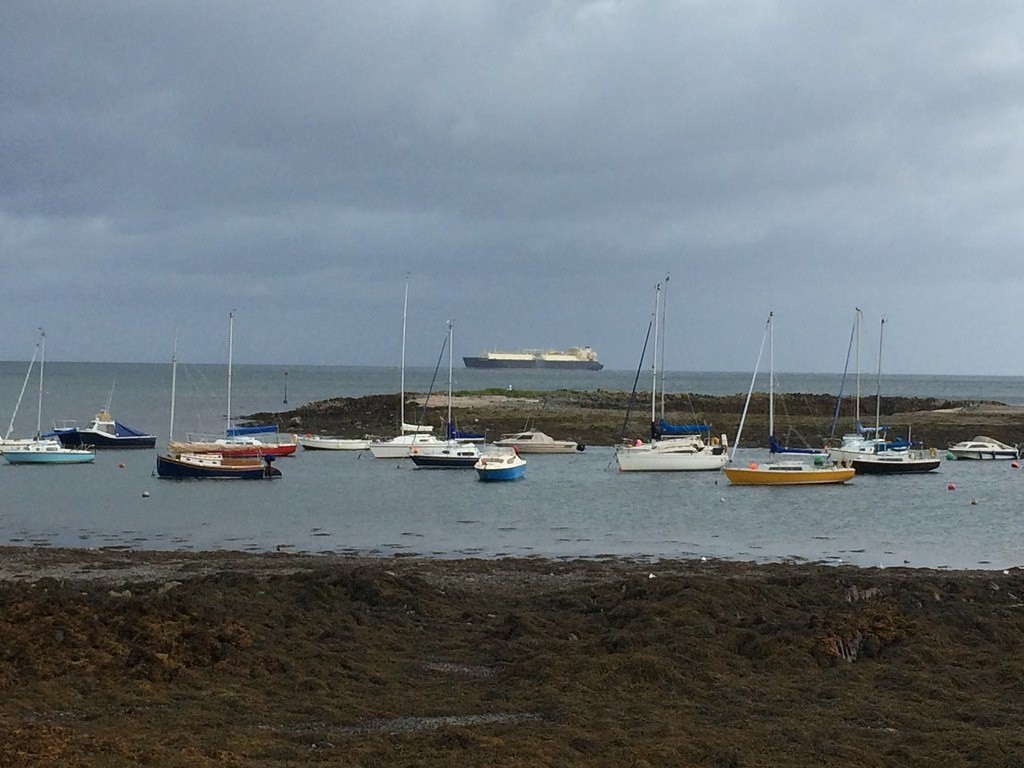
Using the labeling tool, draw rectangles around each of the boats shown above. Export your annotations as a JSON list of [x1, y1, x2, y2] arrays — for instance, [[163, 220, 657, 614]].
[[949, 436, 1019, 460], [293, 430, 375, 451], [463, 345, 604, 370], [491, 413, 579, 453], [157, 338, 282, 477], [37, 377, 156, 449], [475, 445, 526, 479]]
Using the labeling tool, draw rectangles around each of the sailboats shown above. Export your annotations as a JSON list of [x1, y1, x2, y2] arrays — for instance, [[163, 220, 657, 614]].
[[396, 319, 483, 470], [723, 309, 856, 486], [827, 318, 940, 475], [0, 326, 96, 464], [370, 281, 458, 458], [151, 308, 299, 456], [602, 270, 730, 472]]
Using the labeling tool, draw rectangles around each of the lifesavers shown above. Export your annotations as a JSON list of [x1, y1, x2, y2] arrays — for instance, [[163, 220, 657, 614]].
[[577, 442, 585, 451], [930, 449, 937, 458], [291, 434, 298, 444], [711, 437, 719, 445]]
